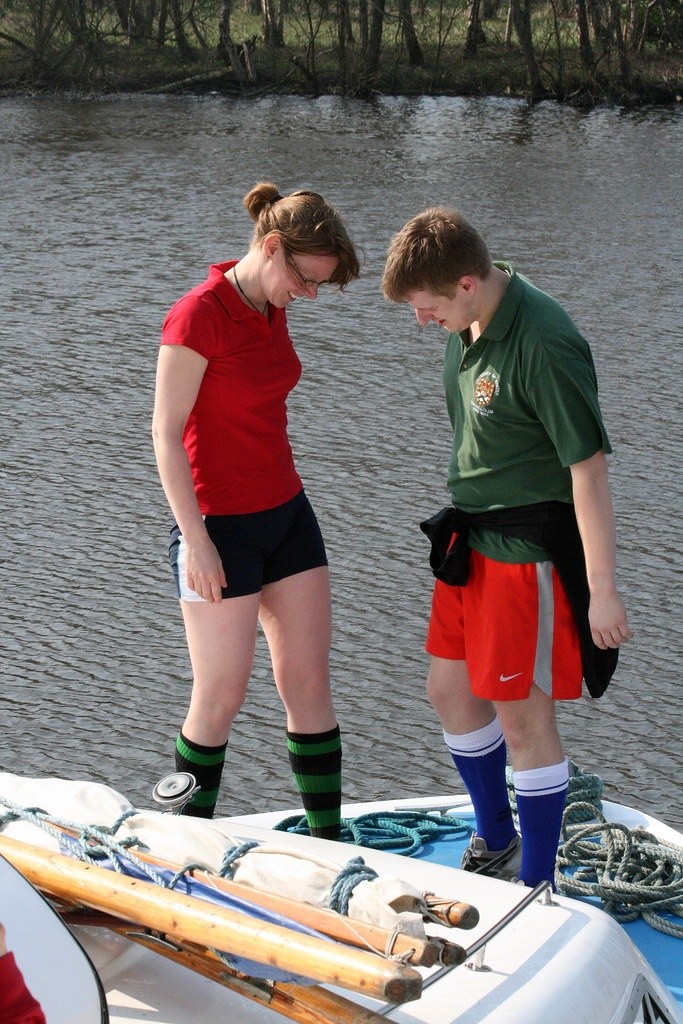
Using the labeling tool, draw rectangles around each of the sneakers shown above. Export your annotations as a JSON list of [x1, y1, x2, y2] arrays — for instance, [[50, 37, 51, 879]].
[[461, 832, 525, 879]]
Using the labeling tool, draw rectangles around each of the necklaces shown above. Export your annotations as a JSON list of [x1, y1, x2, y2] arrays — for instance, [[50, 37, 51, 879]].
[[234, 266, 267, 315]]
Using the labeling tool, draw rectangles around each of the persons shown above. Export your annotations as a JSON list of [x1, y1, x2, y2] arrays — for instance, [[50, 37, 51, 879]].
[[151, 183, 361, 840], [380, 206, 634, 895]]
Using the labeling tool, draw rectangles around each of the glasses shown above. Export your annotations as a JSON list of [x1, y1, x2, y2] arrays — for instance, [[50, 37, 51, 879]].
[[285, 248, 331, 295]]
